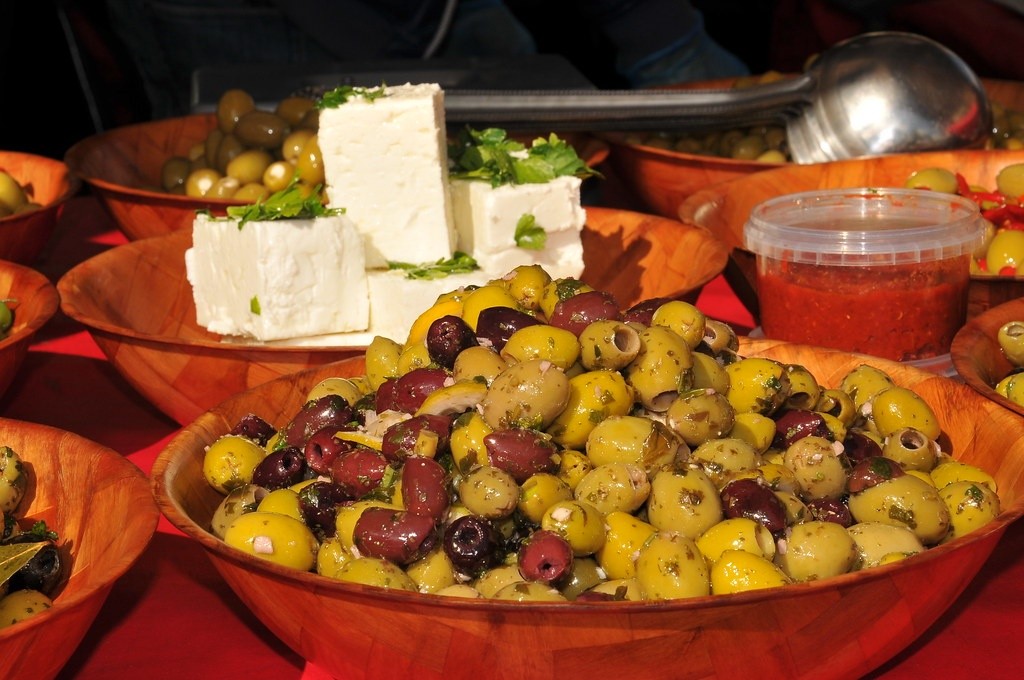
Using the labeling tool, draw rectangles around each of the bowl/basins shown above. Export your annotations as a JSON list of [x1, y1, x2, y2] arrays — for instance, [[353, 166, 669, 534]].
[[949, 295, 1023, 417], [59, 205, 730, 422], [63, 114, 611, 243], [0, 149, 81, 258], [0, 413, 159, 680], [151, 334, 1024, 680], [586, 77, 1024, 218], [677, 147, 1024, 326], [0, 256, 59, 404], [739, 187, 979, 360]]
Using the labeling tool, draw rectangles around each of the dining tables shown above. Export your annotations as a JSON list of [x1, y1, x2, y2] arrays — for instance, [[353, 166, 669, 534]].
[[0, 194, 1024, 680]]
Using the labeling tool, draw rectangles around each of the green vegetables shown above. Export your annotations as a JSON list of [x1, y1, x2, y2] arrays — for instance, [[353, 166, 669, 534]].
[[188, 80, 602, 314]]
